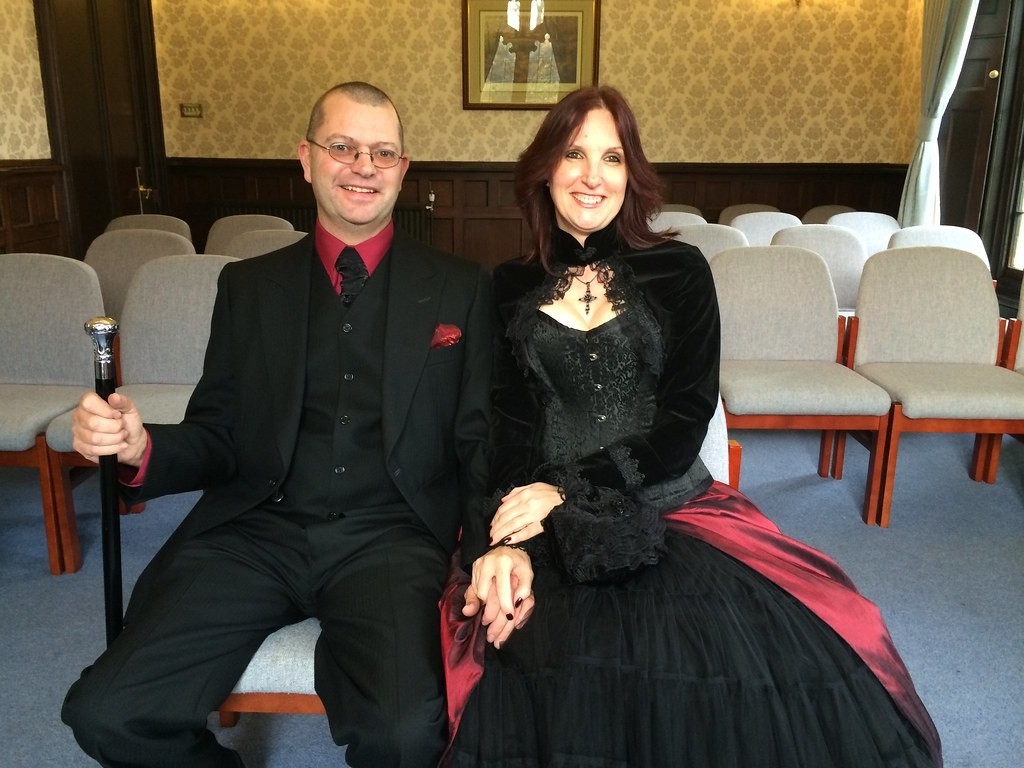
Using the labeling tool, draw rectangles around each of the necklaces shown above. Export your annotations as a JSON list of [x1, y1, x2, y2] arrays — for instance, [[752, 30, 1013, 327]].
[[569, 265, 600, 316]]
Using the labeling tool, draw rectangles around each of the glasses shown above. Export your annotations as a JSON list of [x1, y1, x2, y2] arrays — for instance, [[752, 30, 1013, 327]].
[[308, 139, 404, 168]]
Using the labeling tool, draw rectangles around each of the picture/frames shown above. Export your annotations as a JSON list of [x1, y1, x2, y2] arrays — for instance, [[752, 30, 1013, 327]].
[[462, 1, 601, 110]]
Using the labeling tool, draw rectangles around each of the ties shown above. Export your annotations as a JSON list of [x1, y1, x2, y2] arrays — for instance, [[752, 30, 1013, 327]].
[[336, 246, 369, 308]]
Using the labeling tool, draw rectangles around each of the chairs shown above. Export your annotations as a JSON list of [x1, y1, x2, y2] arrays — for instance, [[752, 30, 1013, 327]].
[[0, 214, 308, 576], [642, 204, 1024, 528], [213, 394, 742, 730]]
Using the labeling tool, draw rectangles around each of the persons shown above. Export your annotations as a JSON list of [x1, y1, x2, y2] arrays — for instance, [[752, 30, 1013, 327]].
[[60, 79, 536, 768], [440, 83, 943, 768]]
